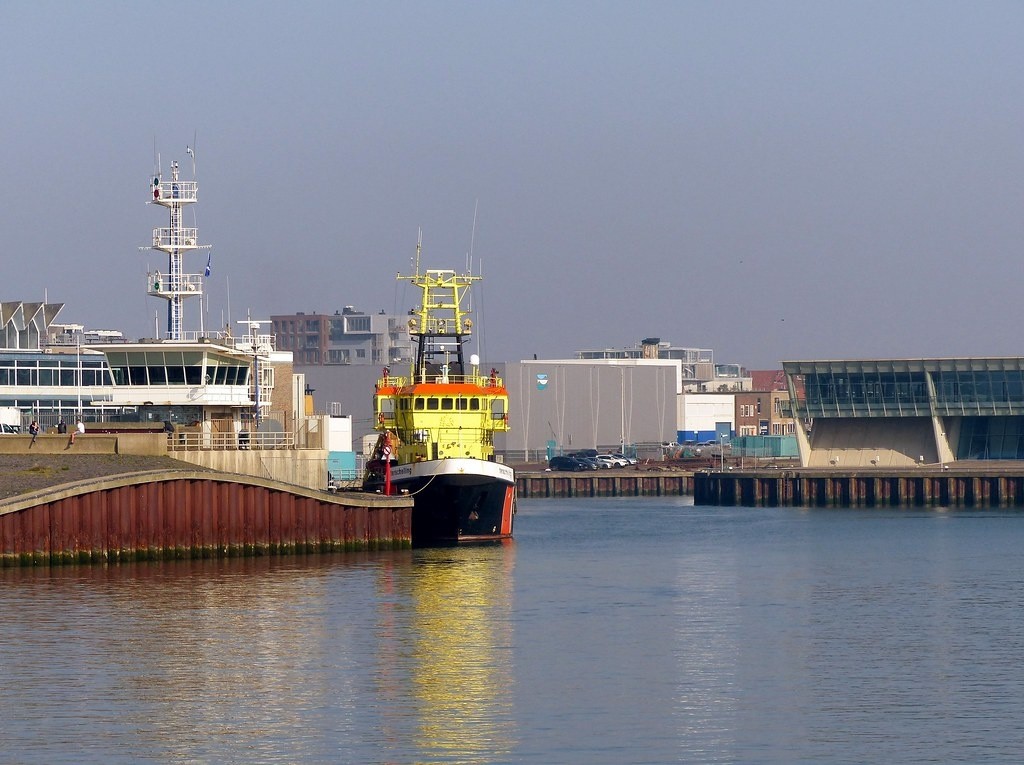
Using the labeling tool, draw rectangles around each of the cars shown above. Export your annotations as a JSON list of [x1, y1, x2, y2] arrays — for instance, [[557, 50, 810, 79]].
[[576, 457, 600, 471], [614, 454, 638, 465], [589, 457, 613, 469]]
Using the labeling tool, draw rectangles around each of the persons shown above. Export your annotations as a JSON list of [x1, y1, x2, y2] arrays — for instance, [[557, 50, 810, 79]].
[[164, 420, 174, 432], [29, 421, 39, 442], [69, 419, 85, 444], [57, 420, 66, 434]]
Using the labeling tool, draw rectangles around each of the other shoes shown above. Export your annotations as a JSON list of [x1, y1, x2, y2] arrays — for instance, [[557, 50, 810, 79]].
[[69, 442, 73, 444], [33, 440, 35, 442]]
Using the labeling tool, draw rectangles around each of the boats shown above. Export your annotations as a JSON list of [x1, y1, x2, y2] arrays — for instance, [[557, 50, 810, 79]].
[[368, 199, 513, 540]]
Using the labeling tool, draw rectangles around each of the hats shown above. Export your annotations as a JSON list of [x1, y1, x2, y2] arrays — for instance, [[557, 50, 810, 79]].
[[61, 419, 64, 422]]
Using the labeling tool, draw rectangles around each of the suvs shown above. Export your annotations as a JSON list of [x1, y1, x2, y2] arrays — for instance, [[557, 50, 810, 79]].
[[549, 456, 588, 472], [596, 454, 630, 468], [569, 448, 599, 456]]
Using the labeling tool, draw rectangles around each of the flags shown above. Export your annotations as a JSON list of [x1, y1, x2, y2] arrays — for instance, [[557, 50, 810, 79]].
[[205, 252, 211, 277]]
